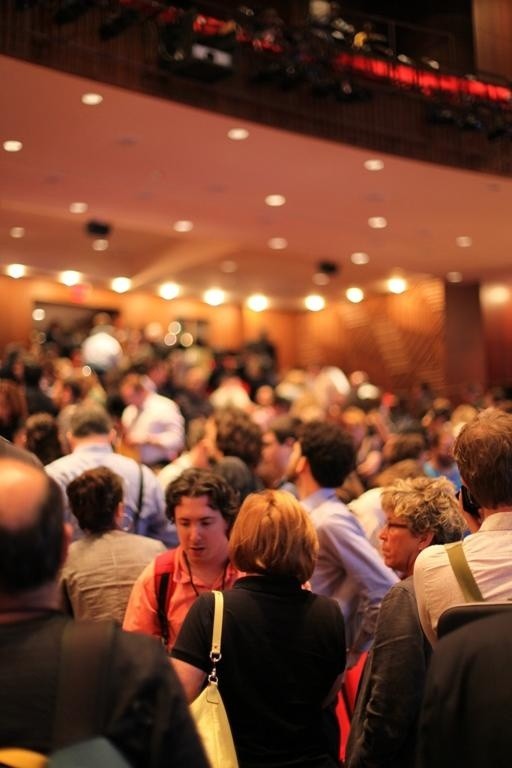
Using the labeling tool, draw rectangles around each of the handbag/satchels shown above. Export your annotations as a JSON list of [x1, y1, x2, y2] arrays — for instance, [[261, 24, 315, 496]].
[[185, 590, 240, 768]]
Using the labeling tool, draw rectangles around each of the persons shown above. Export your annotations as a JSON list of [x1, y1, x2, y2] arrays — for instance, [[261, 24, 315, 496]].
[[2, 313, 512, 768]]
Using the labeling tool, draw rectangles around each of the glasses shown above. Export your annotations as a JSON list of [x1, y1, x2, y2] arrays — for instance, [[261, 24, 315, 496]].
[[380, 518, 411, 533]]
